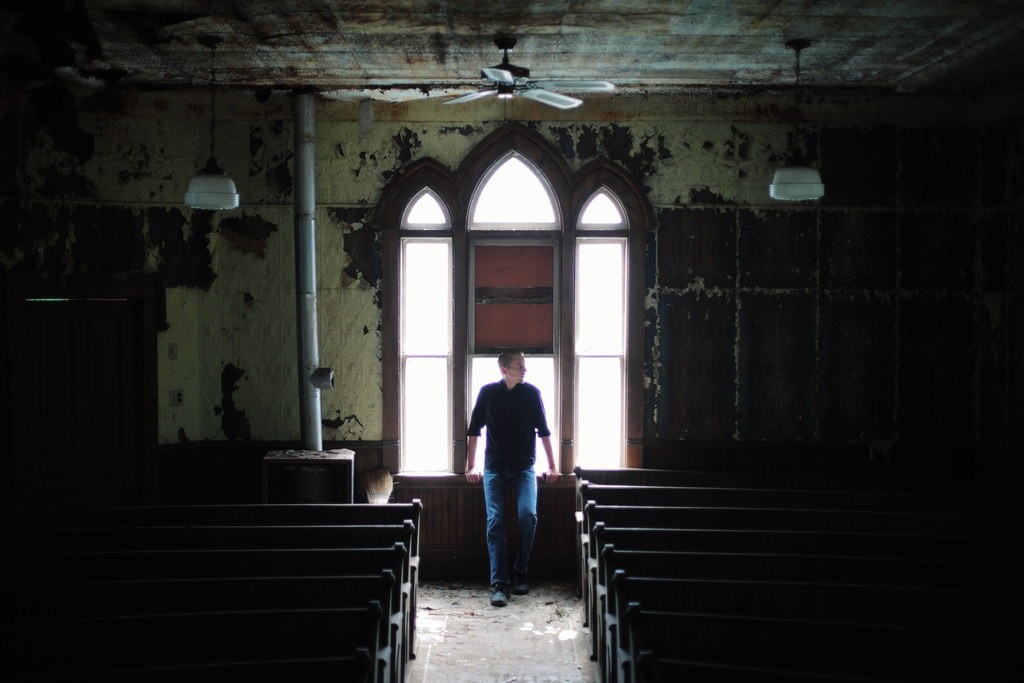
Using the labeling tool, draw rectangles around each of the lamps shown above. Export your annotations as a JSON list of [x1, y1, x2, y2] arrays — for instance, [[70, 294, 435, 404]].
[[184, 35, 240, 210], [769, 36, 826, 201]]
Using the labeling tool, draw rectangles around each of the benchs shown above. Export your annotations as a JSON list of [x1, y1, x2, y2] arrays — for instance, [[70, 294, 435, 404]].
[[0, 497, 424, 683], [575, 484, 1023, 683]]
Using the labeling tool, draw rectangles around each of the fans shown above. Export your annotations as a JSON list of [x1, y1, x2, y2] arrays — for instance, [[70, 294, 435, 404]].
[[440, 33, 585, 110]]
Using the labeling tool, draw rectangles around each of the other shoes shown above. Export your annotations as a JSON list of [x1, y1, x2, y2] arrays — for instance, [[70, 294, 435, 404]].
[[490, 583, 511, 607], [512, 568, 530, 594]]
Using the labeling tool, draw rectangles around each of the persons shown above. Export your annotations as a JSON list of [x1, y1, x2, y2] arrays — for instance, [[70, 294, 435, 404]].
[[464, 348, 558, 607]]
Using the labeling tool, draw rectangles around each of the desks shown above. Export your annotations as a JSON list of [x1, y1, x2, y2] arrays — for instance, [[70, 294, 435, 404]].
[[261, 449, 355, 505]]
